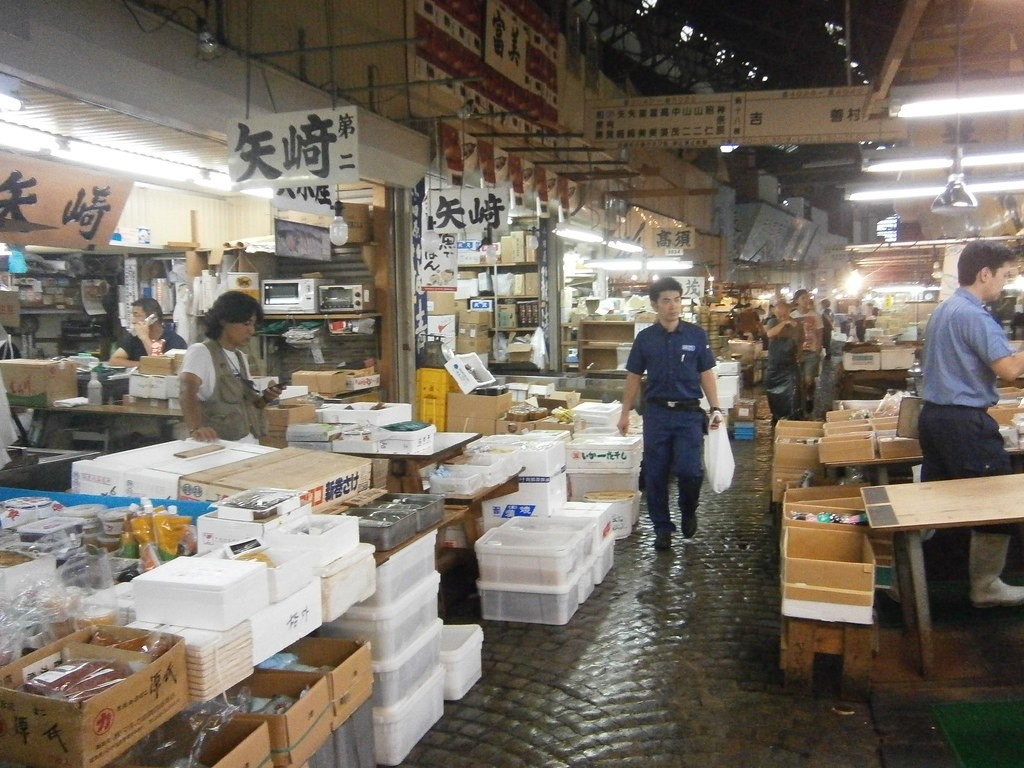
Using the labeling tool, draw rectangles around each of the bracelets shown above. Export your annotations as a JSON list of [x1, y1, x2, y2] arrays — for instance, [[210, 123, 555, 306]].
[[711, 407, 721, 413], [190, 428, 198, 436]]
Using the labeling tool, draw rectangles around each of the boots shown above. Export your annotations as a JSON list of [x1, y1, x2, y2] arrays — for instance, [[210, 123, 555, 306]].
[[881, 527, 935, 603], [970, 530, 1024, 607]]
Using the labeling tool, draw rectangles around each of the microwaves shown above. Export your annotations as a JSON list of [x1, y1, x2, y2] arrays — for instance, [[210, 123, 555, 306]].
[[319, 285, 363, 314], [261, 278, 336, 316]]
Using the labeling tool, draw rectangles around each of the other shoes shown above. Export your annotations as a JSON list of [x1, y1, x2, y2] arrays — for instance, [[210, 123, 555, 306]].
[[787, 409, 803, 421], [824, 354, 832, 360], [770, 413, 780, 427], [805, 397, 814, 414], [654, 529, 671, 550], [681, 511, 697, 537]]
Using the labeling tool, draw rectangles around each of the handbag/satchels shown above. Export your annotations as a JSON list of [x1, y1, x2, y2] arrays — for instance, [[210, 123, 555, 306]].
[[704, 411, 736, 494]]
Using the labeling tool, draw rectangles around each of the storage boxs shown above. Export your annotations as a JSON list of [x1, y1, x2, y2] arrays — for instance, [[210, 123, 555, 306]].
[[0, 231, 1024, 768]]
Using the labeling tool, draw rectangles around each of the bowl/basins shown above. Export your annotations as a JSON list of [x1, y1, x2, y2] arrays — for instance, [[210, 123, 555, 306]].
[[63, 503, 128, 552]]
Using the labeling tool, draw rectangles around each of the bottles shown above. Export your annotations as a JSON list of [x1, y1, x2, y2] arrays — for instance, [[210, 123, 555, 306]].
[[551, 406, 573, 424], [88, 373, 101, 407]]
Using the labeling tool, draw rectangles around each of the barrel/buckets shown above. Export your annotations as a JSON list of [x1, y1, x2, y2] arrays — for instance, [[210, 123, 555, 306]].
[[584, 492, 635, 540]]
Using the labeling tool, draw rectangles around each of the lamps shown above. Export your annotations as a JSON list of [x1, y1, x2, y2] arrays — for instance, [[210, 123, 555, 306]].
[[845, 3, 1023, 217], [552, 221, 643, 253], [330, 202, 348, 246]]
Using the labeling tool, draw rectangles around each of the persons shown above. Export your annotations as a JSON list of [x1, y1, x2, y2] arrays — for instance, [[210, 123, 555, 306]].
[[766, 302, 800, 428], [178, 291, 282, 444], [110, 298, 187, 366], [821, 299, 835, 360], [917, 239, 1024, 606], [764, 305, 776, 324], [618, 277, 723, 550], [852, 302, 865, 341], [789, 289, 824, 419]]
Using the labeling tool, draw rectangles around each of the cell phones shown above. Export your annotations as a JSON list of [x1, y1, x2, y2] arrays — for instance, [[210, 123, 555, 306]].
[[269, 382, 288, 390], [145, 314, 157, 326]]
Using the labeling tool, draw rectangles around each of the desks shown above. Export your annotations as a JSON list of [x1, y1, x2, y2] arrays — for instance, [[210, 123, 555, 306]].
[[860, 473, 1024, 681], [29, 404, 184, 451]]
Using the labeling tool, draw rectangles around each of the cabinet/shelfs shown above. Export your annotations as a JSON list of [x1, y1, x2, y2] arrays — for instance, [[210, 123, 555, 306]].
[[457, 259, 543, 365]]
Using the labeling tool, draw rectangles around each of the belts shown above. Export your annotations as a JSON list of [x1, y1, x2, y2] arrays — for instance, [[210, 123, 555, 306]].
[[647, 396, 700, 411]]
